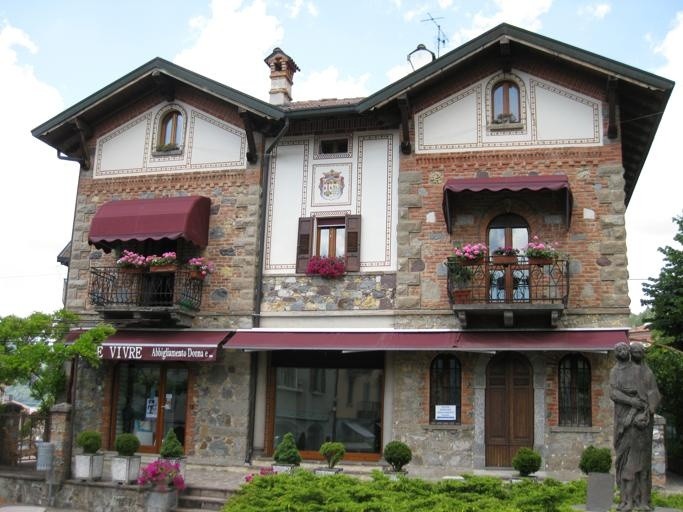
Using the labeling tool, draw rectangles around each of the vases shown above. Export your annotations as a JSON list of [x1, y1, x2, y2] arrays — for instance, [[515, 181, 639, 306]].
[[189, 270, 203, 281], [145, 487, 178, 511], [35, 442, 54, 471]]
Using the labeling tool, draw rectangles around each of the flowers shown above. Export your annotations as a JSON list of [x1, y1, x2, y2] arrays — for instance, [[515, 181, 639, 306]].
[[450, 232, 566, 266], [135, 457, 186, 492], [304, 254, 347, 279], [115, 248, 215, 276]]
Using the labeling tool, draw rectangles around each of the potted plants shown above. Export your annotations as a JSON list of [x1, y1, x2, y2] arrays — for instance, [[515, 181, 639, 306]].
[[451, 266, 474, 305], [73, 427, 185, 485], [510, 446, 542, 487], [269, 430, 412, 480]]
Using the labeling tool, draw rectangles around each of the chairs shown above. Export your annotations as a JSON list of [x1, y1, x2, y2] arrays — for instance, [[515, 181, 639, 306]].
[[2, 427, 36, 466]]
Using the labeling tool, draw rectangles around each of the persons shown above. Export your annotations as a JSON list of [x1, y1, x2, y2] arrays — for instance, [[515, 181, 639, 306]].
[[617, 343, 662, 511], [608, 342, 655, 511]]
